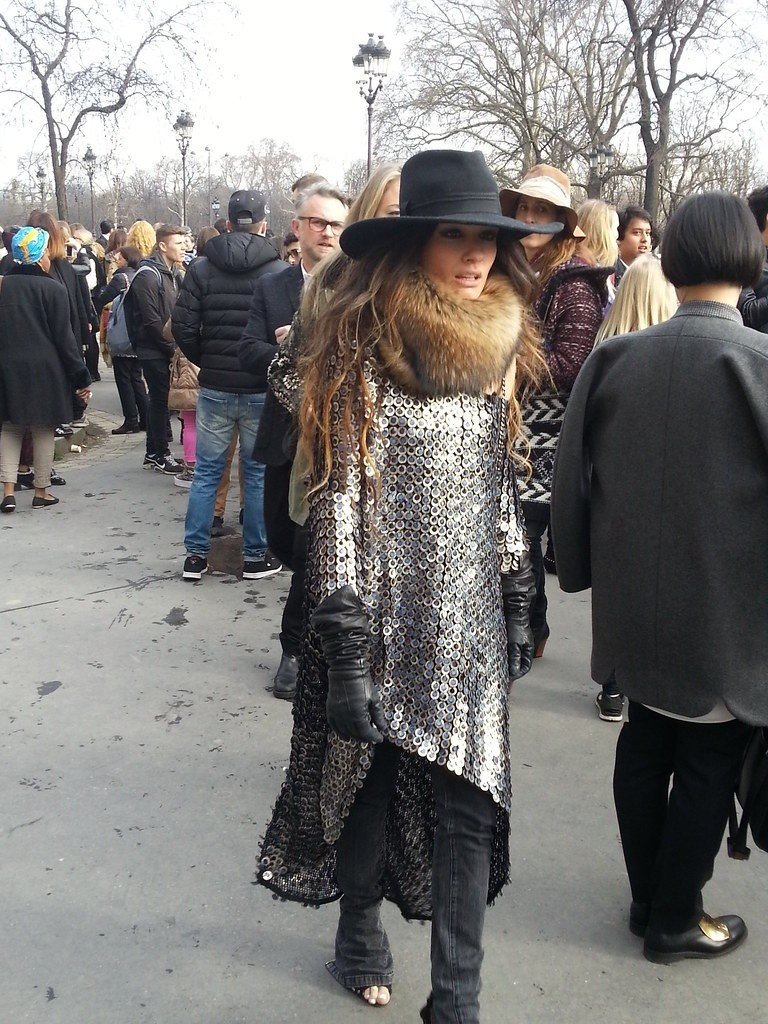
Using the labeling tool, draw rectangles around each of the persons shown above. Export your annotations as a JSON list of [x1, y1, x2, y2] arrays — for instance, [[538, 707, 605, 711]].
[[0, 149, 768, 1024]]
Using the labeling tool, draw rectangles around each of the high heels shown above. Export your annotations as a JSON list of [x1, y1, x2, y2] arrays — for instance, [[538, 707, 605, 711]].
[[50, 467, 66, 484], [15, 467, 36, 491]]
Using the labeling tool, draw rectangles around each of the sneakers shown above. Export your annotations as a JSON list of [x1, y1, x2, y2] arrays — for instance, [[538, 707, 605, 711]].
[[153, 449, 185, 475], [182, 556, 209, 579], [0, 495, 16, 513], [32, 493, 60, 508], [243, 553, 283, 579], [142, 453, 157, 469]]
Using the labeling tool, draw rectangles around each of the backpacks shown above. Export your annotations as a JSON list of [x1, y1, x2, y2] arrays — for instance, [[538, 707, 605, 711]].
[[724, 724, 768, 860], [106, 265, 161, 355]]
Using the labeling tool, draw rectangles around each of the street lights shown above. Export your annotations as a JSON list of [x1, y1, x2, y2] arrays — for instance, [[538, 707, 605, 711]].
[[351, 32, 392, 184], [191, 143, 230, 227], [81, 144, 98, 238], [588, 141, 616, 201], [166, 109, 199, 228]]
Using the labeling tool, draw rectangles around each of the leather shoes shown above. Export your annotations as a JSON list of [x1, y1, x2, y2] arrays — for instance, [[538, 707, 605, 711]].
[[628, 901, 749, 965], [273, 654, 299, 699]]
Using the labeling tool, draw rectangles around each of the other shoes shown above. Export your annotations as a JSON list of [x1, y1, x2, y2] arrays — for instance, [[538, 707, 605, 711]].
[[52, 372, 153, 439], [595, 690, 624, 722], [211, 516, 225, 535], [239, 508, 244, 523], [174, 463, 194, 489], [327, 897, 393, 1007]]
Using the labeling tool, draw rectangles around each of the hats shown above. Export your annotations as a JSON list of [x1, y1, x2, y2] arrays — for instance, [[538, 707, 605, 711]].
[[227, 188, 271, 224], [338, 149, 588, 262], [11, 227, 50, 264]]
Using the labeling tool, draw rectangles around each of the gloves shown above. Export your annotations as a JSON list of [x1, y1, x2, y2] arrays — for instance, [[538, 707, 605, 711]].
[[312, 585, 386, 744], [502, 550, 535, 681]]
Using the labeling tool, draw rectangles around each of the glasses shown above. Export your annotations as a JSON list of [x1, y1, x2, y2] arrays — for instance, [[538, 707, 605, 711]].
[[296, 216, 347, 236]]
